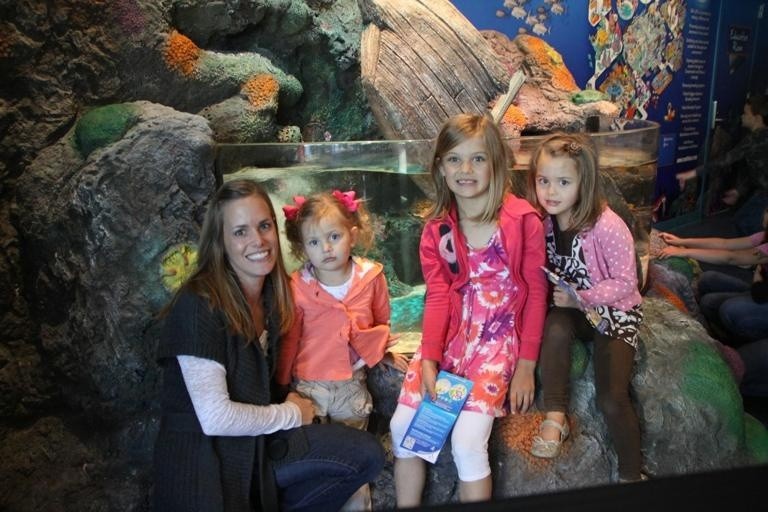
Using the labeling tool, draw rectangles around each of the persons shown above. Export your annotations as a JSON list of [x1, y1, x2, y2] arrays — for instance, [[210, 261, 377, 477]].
[[273, 188, 412, 511], [388, 114, 548, 511], [527, 132, 648, 484], [153, 176, 385, 511], [654, 85, 767, 423]]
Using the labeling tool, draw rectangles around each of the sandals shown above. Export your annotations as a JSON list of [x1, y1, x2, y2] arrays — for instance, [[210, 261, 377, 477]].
[[531, 418, 569, 458]]
